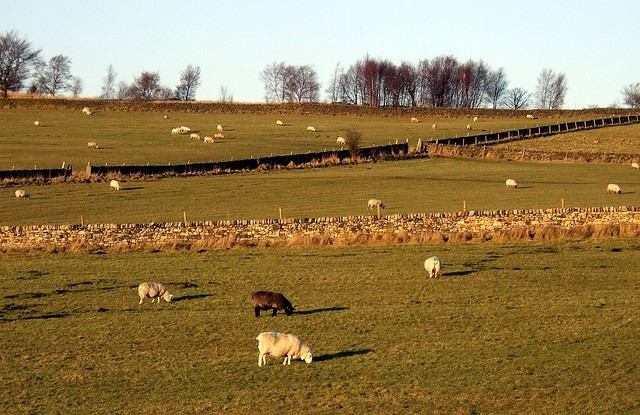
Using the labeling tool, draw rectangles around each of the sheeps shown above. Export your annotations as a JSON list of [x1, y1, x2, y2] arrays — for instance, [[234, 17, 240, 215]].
[[474, 117, 477, 122], [606, 183, 621, 194], [526, 115, 534, 120], [204, 136, 214, 144], [88, 142, 98, 149], [467, 125, 471, 130], [336, 136, 346, 144], [110, 180, 121, 191], [252, 291, 296, 318], [411, 117, 418, 123], [214, 133, 225, 139], [505, 179, 518, 189], [138, 282, 173, 304], [367, 199, 385, 210], [172, 129, 180, 135], [176, 127, 187, 134], [86, 109, 94, 116], [307, 126, 316, 132], [180, 126, 191, 133], [256, 332, 313, 367], [631, 162, 640, 169], [424, 256, 444, 279], [216, 125, 223, 132], [432, 124, 437, 129], [15, 190, 30, 200], [34, 121, 40, 126], [190, 133, 201, 140], [276, 120, 283, 126], [82, 107, 91, 113]]
